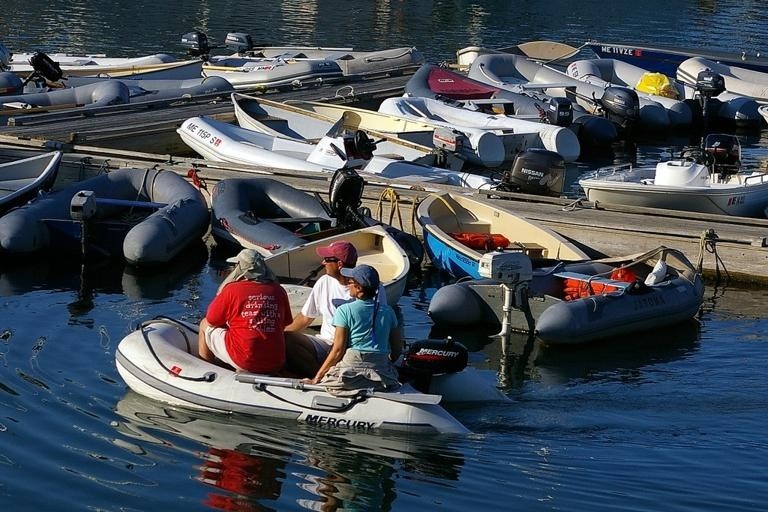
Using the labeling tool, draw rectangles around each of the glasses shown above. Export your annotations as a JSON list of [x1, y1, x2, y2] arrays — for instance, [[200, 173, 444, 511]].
[[322, 254, 344, 265]]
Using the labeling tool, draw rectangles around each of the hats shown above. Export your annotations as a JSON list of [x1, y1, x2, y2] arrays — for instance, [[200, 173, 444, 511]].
[[314, 234, 359, 267], [224, 246, 267, 271], [338, 262, 381, 290]]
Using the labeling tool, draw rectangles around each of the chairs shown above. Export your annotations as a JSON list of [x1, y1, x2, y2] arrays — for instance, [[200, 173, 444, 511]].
[[705, 134, 741, 167]]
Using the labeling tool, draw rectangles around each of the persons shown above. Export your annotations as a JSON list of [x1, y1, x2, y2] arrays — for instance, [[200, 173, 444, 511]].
[[286, 242, 386, 378], [198, 249, 293, 374], [299, 264, 402, 385]]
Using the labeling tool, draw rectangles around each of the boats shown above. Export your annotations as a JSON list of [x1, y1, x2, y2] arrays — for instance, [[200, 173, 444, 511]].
[[111, 388, 448, 460]]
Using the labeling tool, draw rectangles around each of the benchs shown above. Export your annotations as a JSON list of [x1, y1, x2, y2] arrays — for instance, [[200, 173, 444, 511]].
[[506, 242, 549, 258]]
